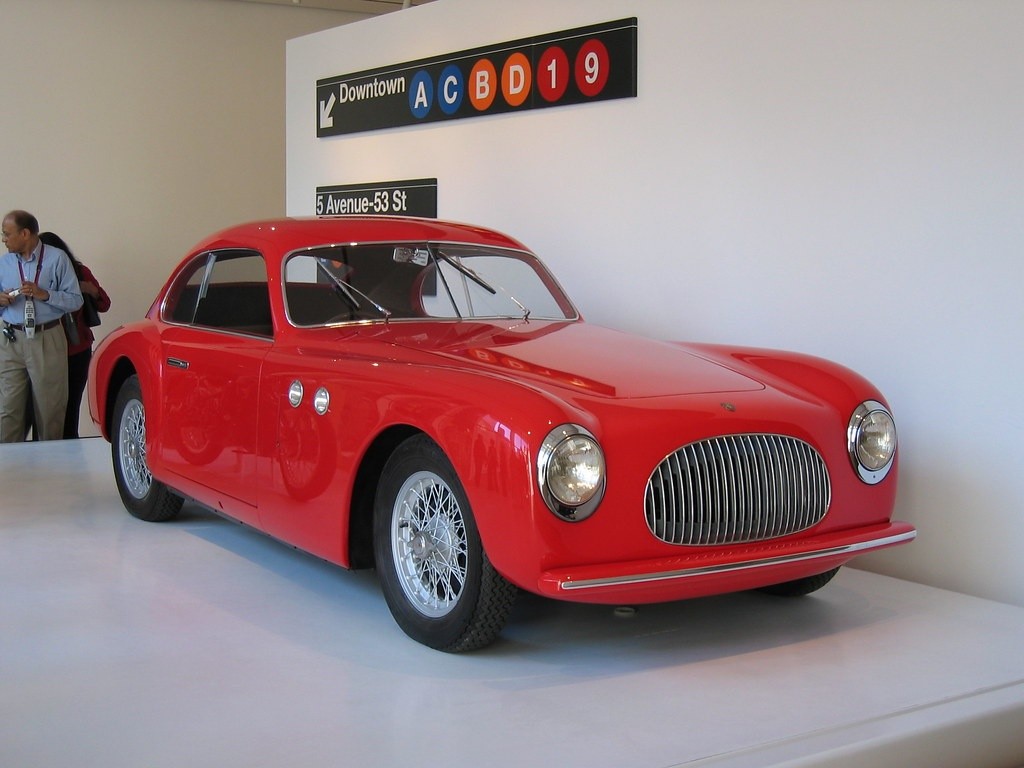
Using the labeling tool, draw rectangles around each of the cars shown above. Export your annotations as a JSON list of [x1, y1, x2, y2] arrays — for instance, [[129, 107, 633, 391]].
[[87, 210, 916, 654]]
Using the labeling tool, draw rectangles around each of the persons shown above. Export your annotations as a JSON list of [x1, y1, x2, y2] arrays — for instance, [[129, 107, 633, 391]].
[[0, 210, 84, 443], [24, 232, 111, 440]]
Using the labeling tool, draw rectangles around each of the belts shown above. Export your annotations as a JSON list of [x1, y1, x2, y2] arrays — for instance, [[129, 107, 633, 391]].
[[3, 319, 60, 331]]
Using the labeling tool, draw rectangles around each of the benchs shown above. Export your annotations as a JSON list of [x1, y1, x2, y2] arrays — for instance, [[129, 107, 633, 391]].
[[178, 282, 348, 336]]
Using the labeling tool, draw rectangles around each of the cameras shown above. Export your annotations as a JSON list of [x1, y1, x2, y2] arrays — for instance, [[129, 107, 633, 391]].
[[8, 288, 21, 297]]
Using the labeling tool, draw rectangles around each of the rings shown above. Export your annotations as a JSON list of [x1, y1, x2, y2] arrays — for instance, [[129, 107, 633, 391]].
[[29, 293, 34, 297], [29, 288, 33, 293]]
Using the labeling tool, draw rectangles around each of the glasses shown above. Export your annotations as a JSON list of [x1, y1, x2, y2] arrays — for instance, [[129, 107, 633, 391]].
[[2, 228, 23, 236]]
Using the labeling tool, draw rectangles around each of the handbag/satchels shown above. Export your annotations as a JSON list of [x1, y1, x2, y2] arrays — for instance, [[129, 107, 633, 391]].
[[84, 293, 101, 326]]
